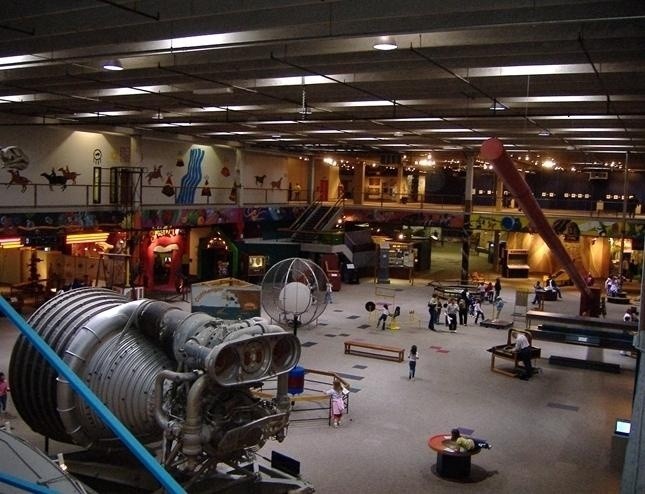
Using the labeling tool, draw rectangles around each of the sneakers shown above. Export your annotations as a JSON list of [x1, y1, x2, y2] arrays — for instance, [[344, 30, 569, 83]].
[[464, 324, 467, 326], [332, 421, 341, 428], [409, 374, 414, 379], [449, 330, 456, 333]]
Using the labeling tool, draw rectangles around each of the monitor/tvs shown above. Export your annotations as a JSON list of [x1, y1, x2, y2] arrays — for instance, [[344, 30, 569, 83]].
[[614, 419, 632, 436], [345, 263, 356, 270]]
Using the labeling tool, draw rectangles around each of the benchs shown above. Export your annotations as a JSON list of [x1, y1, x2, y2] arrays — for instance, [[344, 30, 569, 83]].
[[344, 340, 405, 363]]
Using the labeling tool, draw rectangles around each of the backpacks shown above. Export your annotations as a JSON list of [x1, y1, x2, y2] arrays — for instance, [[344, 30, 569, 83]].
[[459, 298, 466, 309]]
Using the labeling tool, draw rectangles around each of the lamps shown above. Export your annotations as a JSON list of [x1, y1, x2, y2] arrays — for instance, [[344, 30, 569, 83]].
[[103, 59, 124, 72], [372, 36, 398, 51]]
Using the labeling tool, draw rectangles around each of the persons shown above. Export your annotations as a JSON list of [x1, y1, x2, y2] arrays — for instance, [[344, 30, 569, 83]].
[[218, 254, 229, 278], [295, 184, 301, 200], [375, 304, 390, 330], [409, 345, 419, 380], [389, 306, 400, 330], [623, 306, 639, 322], [155, 252, 170, 284], [532, 281, 542, 304], [604, 272, 626, 297], [308, 278, 317, 304], [549, 274, 563, 298], [0, 372, 10, 414], [434, 230, 438, 238], [585, 271, 594, 286], [428, 278, 503, 332], [46, 271, 90, 297], [180, 278, 190, 302], [622, 257, 628, 278], [323, 278, 332, 304], [322, 379, 346, 427], [630, 259, 635, 282], [639, 259, 643, 282], [450, 428, 488, 452], [511, 331, 534, 378]]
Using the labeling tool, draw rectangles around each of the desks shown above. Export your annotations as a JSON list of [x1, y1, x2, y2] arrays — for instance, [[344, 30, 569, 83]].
[[427, 434, 481, 479]]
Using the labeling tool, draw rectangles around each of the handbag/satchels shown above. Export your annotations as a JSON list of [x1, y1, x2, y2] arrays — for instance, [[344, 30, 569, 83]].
[[428, 302, 436, 307]]
